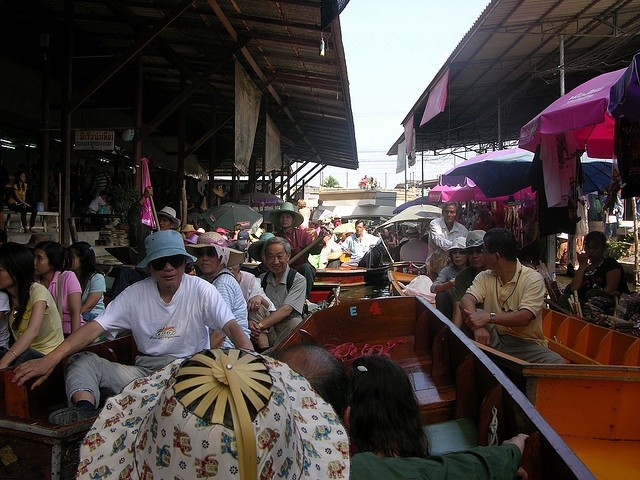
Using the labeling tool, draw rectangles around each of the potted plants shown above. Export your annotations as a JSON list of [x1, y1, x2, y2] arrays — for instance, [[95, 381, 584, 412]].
[[114, 181, 139, 231]]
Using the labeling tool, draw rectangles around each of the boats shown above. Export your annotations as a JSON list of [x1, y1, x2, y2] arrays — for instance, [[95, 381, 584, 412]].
[[309, 269, 434, 302], [450, 298, 640, 480], [0, 286, 134, 480], [246, 294, 596, 479]]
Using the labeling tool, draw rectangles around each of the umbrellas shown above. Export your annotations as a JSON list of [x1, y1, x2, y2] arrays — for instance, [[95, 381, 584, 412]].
[[519, 66, 627, 185], [438, 146, 616, 199], [201, 202, 265, 232], [392, 196, 436, 214], [381, 203, 444, 230], [239, 190, 284, 208], [426, 182, 534, 207], [140, 158, 160, 231], [608, 54, 640, 197]]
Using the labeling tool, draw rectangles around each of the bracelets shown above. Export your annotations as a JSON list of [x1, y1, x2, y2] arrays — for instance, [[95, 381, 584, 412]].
[[257, 322, 266, 332]]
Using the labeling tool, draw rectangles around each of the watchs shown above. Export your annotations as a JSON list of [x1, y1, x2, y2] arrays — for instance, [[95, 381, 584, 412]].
[[490, 311, 496, 324]]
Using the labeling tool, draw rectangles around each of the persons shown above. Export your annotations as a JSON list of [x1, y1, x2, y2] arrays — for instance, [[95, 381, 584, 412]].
[[11, 231, 256, 427], [340, 360, 530, 480], [247, 237, 308, 354], [0, 244, 65, 370], [34, 239, 85, 337], [429, 236, 467, 320], [186, 232, 247, 356], [74, 170, 277, 270], [0, 291, 11, 359], [379, 226, 406, 246], [7, 171, 37, 234], [272, 202, 322, 303], [399, 228, 429, 262], [341, 220, 381, 267], [365, 177, 375, 190], [429, 202, 470, 279], [359, 175, 369, 190], [70, 240, 108, 322], [449, 230, 486, 327], [227, 245, 274, 353], [459, 228, 533, 362], [309, 224, 343, 269], [298, 199, 311, 229], [272, 344, 343, 411]]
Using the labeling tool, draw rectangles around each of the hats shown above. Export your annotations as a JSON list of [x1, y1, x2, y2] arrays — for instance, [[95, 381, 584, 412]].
[[181, 224, 196, 232], [518, 205, 536, 222], [75, 347, 351, 480], [184, 233, 199, 244], [217, 227, 226, 235], [524, 221, 539, 242], [406, 228, 420, 236], [184, 232, 243, 268], [466, 230, 486, 248], [248, 233, 275, 261], [194, 227, 205, 233], [269, 202, 304, 227], [238, 231, 249, 240], [136, 230, 198, 268], [447, 237, 466, 257], [156, 206, 181, 229]]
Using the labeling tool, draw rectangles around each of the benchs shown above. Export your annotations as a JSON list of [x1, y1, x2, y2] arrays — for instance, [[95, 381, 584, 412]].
[[3, 209, 61, 233]]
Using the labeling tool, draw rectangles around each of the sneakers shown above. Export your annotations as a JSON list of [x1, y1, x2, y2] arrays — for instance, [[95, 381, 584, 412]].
[[49, 407, 99, 424]]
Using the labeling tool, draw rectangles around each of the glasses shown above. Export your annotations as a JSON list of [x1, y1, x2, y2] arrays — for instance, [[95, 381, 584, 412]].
[[194, 248, 217, 257], [451, 250, 465, 255], [468, 249, 484, 253], [150, 255, 184, 270], [12, 306, 19, 330]]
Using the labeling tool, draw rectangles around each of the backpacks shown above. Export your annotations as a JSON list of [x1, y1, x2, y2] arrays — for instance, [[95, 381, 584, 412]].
[[261, 268, 308, 320]]
